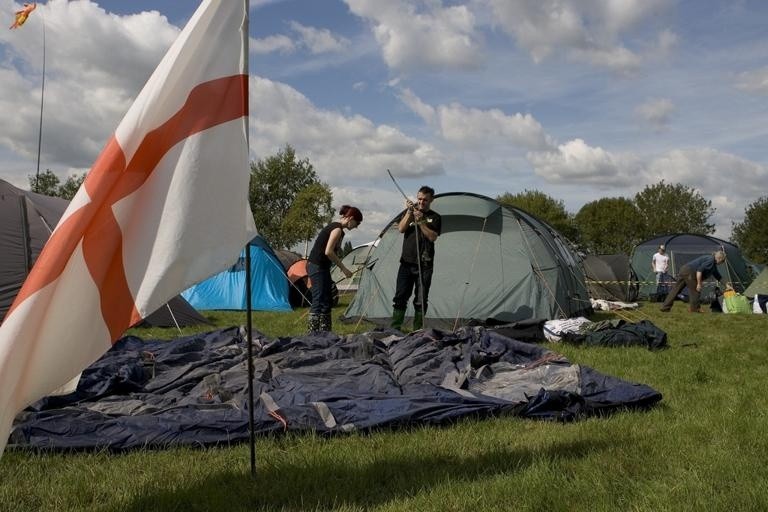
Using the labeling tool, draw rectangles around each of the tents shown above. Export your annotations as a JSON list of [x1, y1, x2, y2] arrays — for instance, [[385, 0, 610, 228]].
[[1, 178, 219, 329], [271, 247, 339, 308], [339, 190, 595, 342], [629, 232, 754, 301], [583, 254, 640, 303], [330, 240, 381, 291], [180, 230, 294, 313], [742, 265, 768, 297], [269, 241, 302, 276]]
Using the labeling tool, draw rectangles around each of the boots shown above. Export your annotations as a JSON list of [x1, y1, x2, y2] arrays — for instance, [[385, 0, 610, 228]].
[[307, 313, 319, 335], [319, 313, 332, 333], [413, 310, 425, 332], [390, 308, 404, 331]]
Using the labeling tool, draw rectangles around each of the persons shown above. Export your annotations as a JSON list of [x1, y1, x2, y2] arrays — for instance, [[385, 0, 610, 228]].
[[651, 245, 670, 302], [660, 250, 734, 313], [390, 186, 441, 331], [305, 205, 363, 333]]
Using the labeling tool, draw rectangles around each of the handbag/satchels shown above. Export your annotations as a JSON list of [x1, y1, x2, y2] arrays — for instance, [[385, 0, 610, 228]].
[[710, 286, 753, 317]]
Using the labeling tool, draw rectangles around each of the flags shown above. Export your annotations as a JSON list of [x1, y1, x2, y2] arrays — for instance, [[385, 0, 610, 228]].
[[0, 1, 258, 458], [8, 3, 37, 31]]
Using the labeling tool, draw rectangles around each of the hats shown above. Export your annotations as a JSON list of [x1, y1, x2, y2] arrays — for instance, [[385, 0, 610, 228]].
[[658, 244, 666, 251]]
